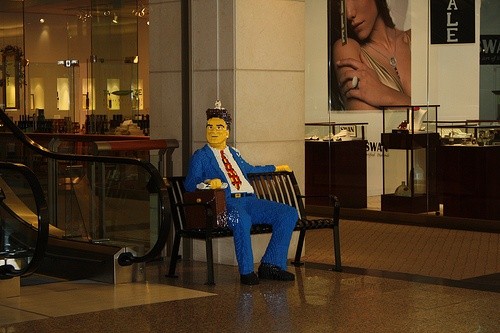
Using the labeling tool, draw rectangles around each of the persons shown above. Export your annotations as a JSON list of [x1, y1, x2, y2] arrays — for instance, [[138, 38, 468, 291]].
[[330, 0, 411, 110], [182, 108, 299, 286]]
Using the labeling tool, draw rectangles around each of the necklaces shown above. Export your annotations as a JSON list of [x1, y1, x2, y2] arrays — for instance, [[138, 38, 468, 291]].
[[364, 27, 401, 80]]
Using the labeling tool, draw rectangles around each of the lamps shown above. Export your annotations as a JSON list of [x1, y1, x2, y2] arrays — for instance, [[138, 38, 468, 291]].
[[112, 12, 120, 23]]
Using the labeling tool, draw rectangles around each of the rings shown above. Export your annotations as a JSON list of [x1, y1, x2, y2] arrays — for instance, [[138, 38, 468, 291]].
[[352, 75, 357, 88]]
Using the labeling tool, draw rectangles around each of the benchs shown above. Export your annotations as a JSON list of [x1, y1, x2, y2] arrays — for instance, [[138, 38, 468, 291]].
[[163, 171, 343, 285]]
